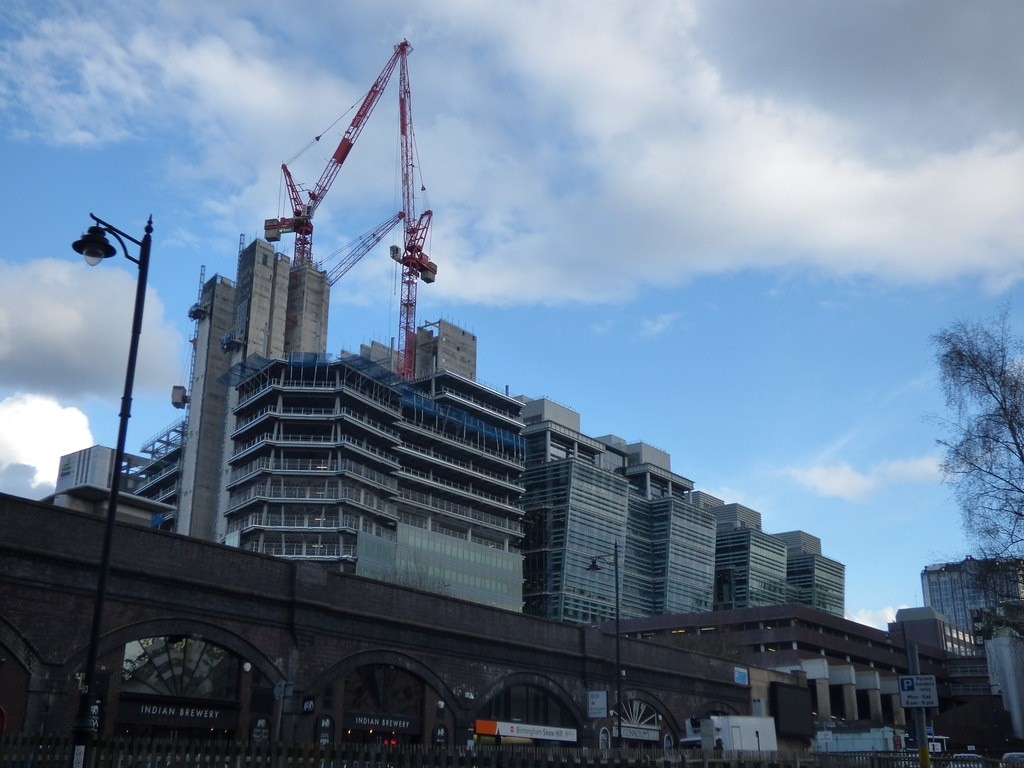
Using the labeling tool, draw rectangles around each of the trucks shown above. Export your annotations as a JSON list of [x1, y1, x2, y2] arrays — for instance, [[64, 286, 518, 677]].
[[678, 714, 777, 754]]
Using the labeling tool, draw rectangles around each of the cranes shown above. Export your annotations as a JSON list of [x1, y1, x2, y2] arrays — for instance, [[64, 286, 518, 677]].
[[263, 37, 437, 385]]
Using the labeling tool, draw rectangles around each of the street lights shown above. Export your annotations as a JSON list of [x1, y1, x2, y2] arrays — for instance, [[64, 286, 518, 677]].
[[69, 213, 158, 768], [585, 539, 622, 752]]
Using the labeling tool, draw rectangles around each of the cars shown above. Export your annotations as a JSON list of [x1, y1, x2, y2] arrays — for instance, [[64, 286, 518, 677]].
[[945, 753, 986, 768], [1000, 753, 1024, 768]]
